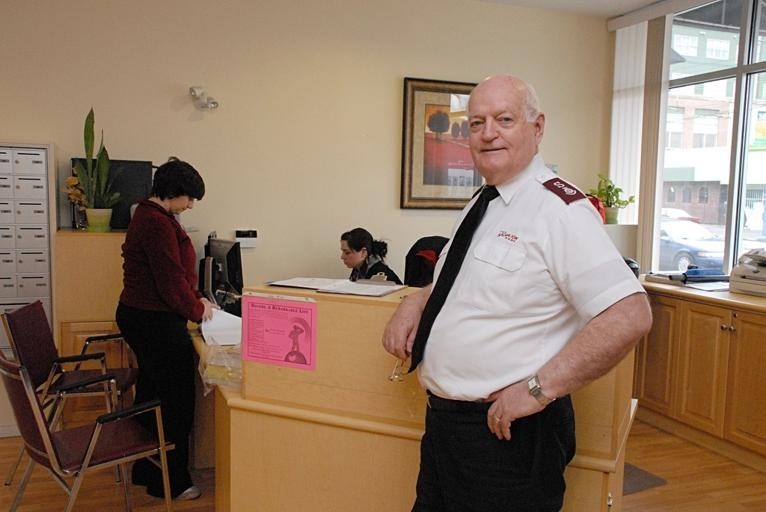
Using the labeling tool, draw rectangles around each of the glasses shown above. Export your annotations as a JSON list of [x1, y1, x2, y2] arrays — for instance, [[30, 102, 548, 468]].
[[389, 359, 403, 382]]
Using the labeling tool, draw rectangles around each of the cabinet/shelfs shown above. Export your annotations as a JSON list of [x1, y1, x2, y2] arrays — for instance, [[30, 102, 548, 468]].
[[632, 273, 766, 474], [0, 142, 63, 438]]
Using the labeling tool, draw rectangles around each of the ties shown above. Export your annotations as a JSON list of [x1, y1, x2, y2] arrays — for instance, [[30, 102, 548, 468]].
[[405, 184, 500, 374]]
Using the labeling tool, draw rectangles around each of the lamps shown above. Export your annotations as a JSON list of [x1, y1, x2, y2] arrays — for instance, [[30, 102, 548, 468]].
[[189, 85, 219, 112]]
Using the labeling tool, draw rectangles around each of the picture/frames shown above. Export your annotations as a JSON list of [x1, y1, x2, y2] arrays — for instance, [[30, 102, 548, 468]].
[[400, 76, 488, 209]]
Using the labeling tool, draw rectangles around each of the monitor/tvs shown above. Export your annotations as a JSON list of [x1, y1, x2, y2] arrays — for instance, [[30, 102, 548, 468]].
[[199, 236, 243, 317]]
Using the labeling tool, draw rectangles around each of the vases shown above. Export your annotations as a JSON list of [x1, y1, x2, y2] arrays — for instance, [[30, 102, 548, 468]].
[[71, 203, 87, 231]]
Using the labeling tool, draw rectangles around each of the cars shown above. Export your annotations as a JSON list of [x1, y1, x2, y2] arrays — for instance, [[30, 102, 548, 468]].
[[659, 215, 725, 275], [661, 206, 702, 224]]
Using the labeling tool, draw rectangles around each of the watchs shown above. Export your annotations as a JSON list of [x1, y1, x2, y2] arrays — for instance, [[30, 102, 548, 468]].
[[525, 374, 556, 408]]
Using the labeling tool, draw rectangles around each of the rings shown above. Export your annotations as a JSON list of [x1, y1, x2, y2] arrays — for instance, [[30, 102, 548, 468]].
[[492, 413, 502, 424]]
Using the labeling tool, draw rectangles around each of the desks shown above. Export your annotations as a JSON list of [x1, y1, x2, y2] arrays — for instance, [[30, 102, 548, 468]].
[[185, 283, 244, 472]]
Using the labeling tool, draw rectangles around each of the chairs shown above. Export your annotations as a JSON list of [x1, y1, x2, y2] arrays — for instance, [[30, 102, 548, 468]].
[[0, 349, 176, 512], [0, 299, 139, 485]]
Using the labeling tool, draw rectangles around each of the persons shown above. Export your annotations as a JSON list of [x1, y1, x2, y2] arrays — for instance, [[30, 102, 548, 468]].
[[338, 229, 401, 284], [113, 157, 220, 501], [382, 73, 656, 511]]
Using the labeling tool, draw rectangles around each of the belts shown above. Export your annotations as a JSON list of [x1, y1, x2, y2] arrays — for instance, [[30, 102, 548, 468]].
[[425, 389, 492, 413]]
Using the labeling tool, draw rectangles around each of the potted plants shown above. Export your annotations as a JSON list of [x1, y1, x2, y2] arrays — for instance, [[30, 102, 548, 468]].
[[73, 106, 123, 233], [589, 175, 636, 224]]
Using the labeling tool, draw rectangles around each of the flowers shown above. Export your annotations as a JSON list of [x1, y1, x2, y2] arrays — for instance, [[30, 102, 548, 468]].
[[61, 177, 88, 208]]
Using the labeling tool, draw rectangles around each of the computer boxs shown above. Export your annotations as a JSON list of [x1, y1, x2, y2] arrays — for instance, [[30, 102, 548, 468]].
[[71, 157, 154, 230]]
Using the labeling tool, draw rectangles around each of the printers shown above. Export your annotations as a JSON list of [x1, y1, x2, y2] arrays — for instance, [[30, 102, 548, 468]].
[[730, 249, 766, 298]]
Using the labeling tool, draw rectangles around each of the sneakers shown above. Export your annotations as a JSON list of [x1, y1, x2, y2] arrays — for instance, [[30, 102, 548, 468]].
[[176, 486, 200, 500]]
